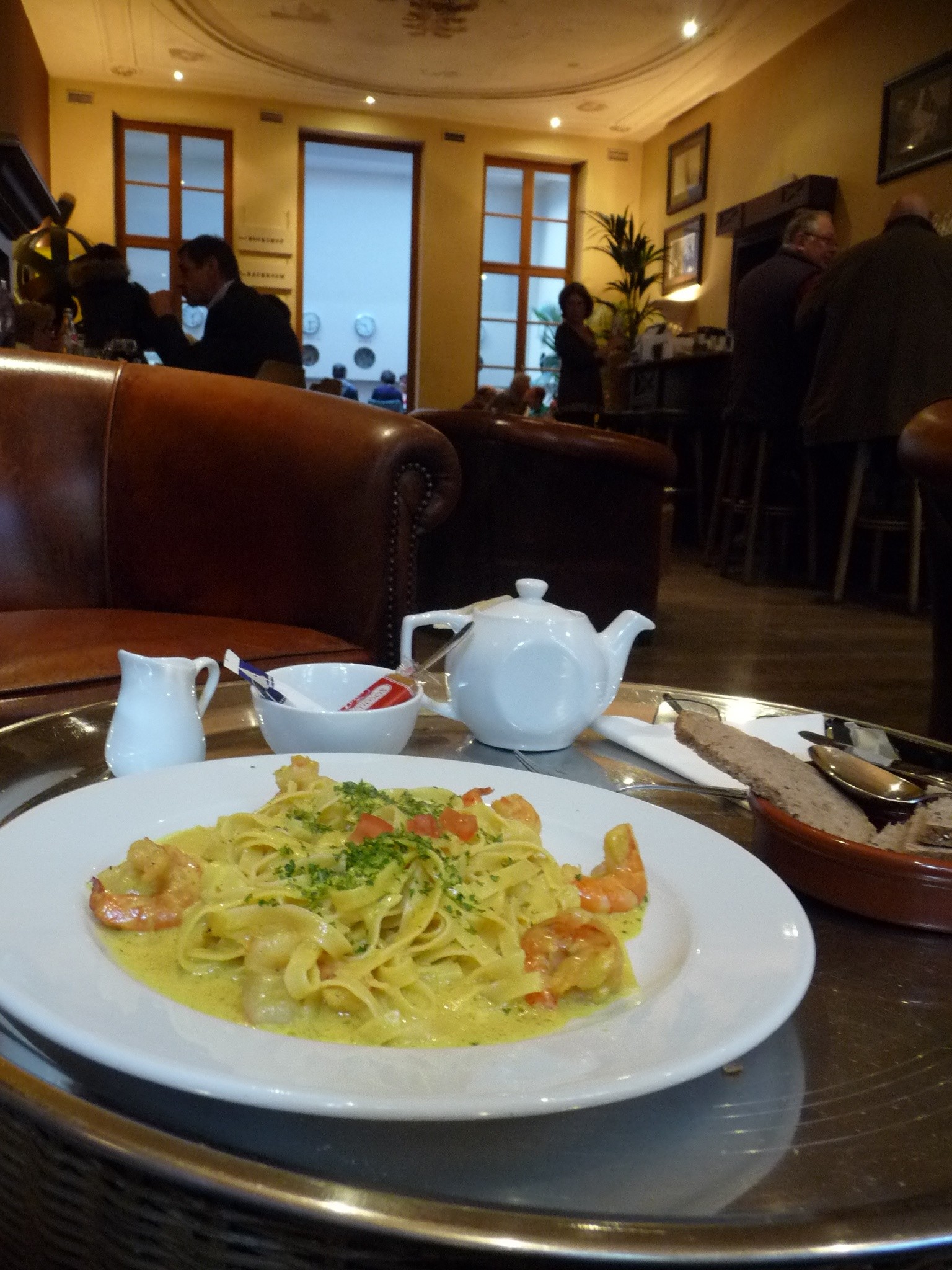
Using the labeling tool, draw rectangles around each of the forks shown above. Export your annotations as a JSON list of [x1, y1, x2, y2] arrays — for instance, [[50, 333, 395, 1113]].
[[515, 750, 747, 798]]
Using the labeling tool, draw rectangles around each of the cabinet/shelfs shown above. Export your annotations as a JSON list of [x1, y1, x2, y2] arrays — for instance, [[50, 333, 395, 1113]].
[[617, 327, 733, 548]]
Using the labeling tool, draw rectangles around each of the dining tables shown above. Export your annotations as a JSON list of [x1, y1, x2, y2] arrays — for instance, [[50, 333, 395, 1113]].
[[0, 673, 952, 1270]]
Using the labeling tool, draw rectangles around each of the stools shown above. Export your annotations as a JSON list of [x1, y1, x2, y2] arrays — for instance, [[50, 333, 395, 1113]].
[[705, 427, 817, 585], [833, 436, 922, 614]]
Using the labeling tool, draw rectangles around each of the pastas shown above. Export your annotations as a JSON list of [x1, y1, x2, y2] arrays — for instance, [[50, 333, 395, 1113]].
[[176, 777, 588, 1036]]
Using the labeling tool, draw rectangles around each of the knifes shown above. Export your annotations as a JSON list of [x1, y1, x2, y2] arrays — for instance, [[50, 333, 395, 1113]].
[[798, 730, 952, 790]]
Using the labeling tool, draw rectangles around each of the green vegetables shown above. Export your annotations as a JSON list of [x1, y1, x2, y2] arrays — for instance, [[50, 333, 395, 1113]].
[[239, 779, 516, 956]]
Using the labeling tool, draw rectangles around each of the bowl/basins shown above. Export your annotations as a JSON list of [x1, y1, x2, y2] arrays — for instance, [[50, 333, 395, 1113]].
[[250, 663, 424, 755]]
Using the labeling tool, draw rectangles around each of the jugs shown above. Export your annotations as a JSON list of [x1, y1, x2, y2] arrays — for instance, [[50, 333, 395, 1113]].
[[104, 649, 220, 777]]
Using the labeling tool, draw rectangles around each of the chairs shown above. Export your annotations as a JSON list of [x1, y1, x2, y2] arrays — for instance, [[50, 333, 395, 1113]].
[[368, 399, 404, 414], [321, 378, 342, 395]]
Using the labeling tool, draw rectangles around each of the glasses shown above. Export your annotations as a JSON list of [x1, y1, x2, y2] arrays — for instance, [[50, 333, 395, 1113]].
[[804, 232, 836, 246]]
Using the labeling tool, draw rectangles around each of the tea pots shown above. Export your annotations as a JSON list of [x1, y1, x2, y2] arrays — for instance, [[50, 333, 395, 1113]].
[[401, 578, 656, 751]]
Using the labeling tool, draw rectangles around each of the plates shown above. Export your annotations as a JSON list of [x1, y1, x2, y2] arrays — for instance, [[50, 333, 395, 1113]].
[[0, 754, 815, 1121], [746, 788, 952, 932]]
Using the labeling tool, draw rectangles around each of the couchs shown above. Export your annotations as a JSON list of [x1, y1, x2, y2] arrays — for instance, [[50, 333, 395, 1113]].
[[409, 409, 676, 646], [0, 351, 463, 730]]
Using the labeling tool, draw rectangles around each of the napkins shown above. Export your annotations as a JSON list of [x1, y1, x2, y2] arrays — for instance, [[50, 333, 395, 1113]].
[[589, 714, 826, 790]]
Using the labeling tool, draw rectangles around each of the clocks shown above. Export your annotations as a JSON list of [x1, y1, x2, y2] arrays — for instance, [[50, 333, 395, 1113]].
[[302, 312, 320, 333], [355, 315, 376, 337]]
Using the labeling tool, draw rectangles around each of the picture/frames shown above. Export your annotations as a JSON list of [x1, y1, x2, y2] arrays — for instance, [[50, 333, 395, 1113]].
[[875, 49, 952, 187], [662, 211, 703, 298], [665, 122, 711, 214]]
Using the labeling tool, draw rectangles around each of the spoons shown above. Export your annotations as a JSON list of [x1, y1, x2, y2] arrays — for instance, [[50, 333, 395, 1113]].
[[808, 745, 952, 804]]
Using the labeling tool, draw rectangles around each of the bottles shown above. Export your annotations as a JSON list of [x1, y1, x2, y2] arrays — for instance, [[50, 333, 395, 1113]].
[[59, 308, 78, 355]]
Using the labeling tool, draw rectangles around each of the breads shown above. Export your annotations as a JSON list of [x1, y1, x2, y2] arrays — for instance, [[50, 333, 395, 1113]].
[[674, 710, 952, 861]]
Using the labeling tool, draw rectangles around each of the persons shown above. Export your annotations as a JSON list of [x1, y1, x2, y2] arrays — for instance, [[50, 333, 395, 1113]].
[[794, 194, 952, 609], [461, 355, 561, 418], [555, 281, 622, 425], [333, 364, 358, 400], [75, 244, 153, 365], [150, 235, 305, 388], [398, 375, 410, 408], [372, 371, 403, 406], [734, 209, 835, 589]]
